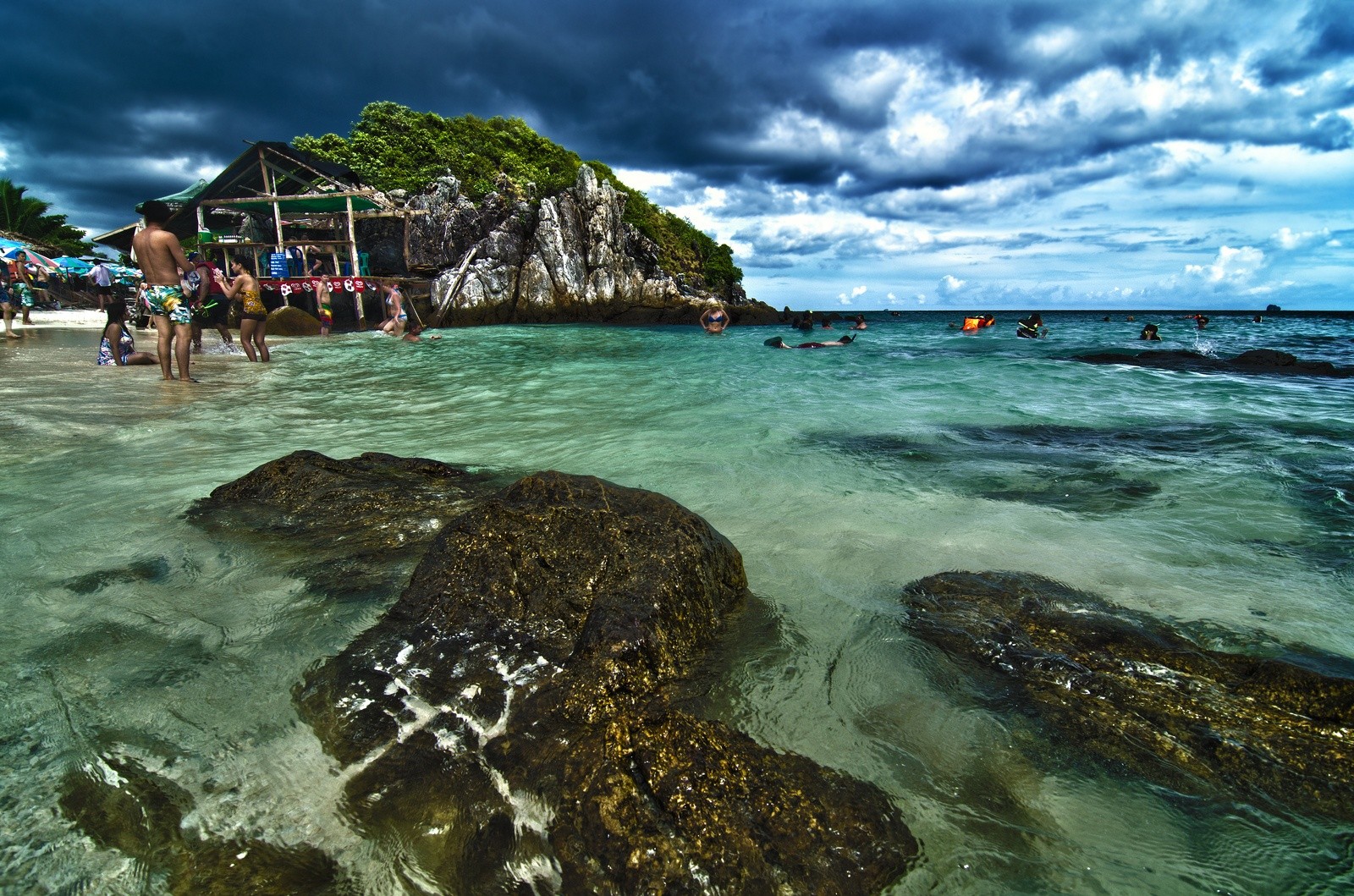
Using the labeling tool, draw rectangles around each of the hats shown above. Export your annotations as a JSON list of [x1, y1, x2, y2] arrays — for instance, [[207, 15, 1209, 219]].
[[188, 251, 199, 261]]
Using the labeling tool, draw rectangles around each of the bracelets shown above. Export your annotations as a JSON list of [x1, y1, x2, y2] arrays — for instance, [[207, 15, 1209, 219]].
[[190, 262, 196, 270]]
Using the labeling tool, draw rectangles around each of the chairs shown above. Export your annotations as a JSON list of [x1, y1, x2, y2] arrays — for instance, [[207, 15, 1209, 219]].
[[343, 248, 362, 276], [358, 253, 370, 276], [286, 246, 316, 276]]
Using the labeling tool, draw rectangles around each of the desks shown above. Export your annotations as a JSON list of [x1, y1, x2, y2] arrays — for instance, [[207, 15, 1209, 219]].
[[308, 250, 341, 276]]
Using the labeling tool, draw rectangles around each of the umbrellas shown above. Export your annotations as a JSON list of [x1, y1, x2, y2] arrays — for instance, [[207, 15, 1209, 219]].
[[0, 237, 143, 285]]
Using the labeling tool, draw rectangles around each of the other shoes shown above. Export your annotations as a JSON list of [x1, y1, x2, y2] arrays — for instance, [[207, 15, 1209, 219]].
[[764, 335, 783, 346]]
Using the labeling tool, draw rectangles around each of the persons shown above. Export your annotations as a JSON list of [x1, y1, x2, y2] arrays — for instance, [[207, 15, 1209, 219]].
[[188, 252, 233, 355], [33, 264, 51, 310], [1197, 317, 1209, 330], [0, 256, 21, 337], [699, 300, 730, 333], [849, 314, 867, 330], [96, 300, 195, 366], [820, 318, 833, 329], [284, 234, 322, 276], [1252, 316, 1263, 323], [764, 333, 857, 349], [316, 271, 333, 337], [132, 200, 199, 382], [381, 279, 407, 336], [88, 258, 116, 313], [214, 253, 270, 362], [403, 321, 442, 342], [799, 310, 813, 330], [985, 314, 995, 325], [256, 241, 273, 268], [792, 316, 802, 328], [135, 278, 158, 330], [378, 287, 404, 329]]
